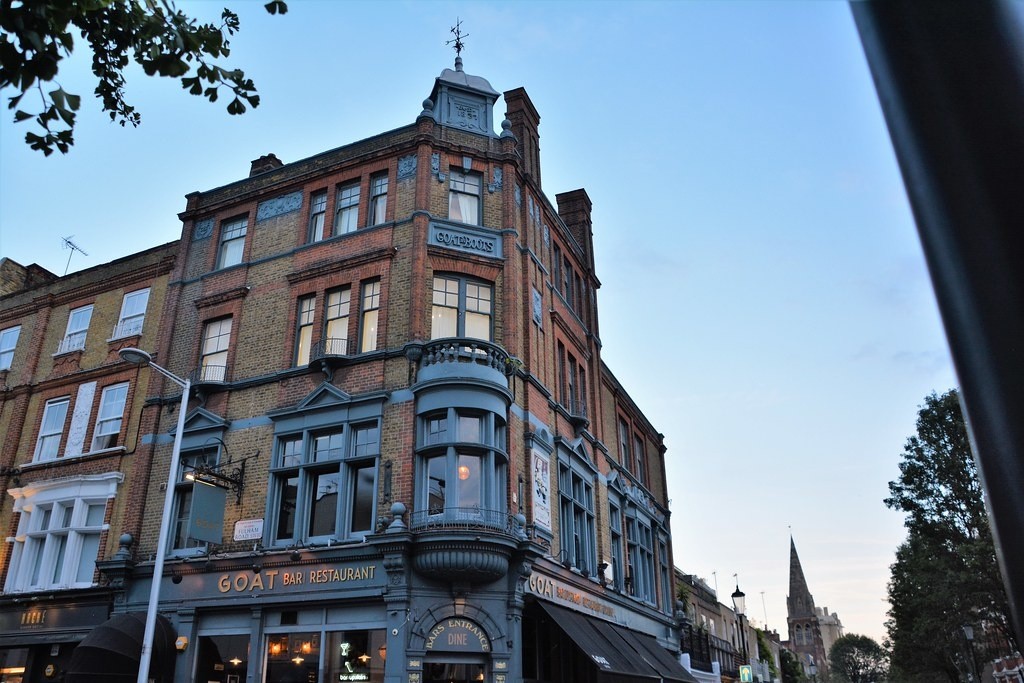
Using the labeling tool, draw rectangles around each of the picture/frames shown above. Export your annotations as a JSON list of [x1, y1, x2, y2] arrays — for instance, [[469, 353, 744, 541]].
[[228, 675, 239, 683], [311, 634, 319, 648]]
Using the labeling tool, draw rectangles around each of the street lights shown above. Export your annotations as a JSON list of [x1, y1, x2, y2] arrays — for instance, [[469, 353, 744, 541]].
[[731, 585, 748, 665], [117, 347, 190, 683]]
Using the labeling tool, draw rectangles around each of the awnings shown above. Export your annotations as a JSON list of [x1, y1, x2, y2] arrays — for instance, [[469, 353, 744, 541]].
[[524, 592, 700, 683], [65, 610, 177, 683]]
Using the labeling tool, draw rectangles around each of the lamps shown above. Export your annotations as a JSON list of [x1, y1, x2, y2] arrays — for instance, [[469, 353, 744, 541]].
[[291, 540, 316, 562], [609, 578, 621, 596], [185, 436, 231, 488], [249, 552, 265, 572], [171, 562, 183, 584], [546, 550, 571, 569], [205, 550, 226, 567], [576, 560, 589, 577]]
